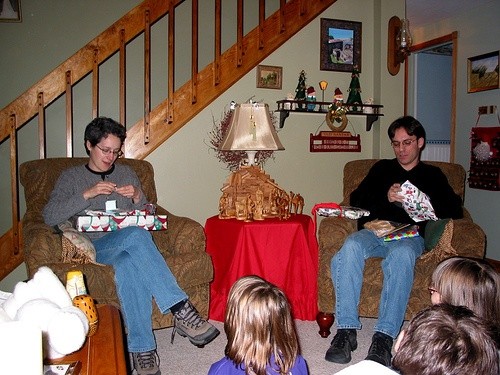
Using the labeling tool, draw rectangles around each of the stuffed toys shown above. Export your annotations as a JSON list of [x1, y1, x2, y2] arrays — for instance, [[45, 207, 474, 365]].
[[13, 265, 89, 361]]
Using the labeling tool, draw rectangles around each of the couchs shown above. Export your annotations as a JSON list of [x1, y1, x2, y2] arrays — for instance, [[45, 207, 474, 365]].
[[19, 157, 213, 333], [317, 159, 486, 338]]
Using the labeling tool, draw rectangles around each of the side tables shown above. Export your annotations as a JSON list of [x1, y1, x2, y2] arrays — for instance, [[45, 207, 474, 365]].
[[204, 214, 319, 323]]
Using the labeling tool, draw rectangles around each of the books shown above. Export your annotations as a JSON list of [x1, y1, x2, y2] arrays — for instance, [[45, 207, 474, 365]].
[[316, 206, 371, 216], [383, 224, 420, 242], [364, 217, 411, 239]]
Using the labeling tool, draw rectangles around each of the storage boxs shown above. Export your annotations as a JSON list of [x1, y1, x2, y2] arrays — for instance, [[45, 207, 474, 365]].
[[72, 215, 168, 232]]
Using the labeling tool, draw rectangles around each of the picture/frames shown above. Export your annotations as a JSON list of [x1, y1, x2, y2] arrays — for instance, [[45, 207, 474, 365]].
[[256, 65, 282, 89], [0, 0, 23, 23], [467, 50, 500, 94], [320, 18, 362, 73]]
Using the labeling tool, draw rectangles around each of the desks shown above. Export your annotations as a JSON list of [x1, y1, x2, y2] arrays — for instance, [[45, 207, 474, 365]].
[[43, 304, 127, 375]]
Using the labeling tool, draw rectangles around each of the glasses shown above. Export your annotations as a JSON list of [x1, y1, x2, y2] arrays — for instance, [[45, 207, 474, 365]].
[[96, 145, 124, 157], [428, 285, 438, 293], [391, 139, 416, 148]]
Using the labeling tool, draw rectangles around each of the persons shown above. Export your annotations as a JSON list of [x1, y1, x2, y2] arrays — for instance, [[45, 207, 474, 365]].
[[431, 256, 500, 343], [346, 70, 363, 105], [43, 116, 220, 375], [324, 115, 463, 368], [208, 276, 309, 375], [307, 87, 316, 110], [333, 88, 343, 107], [331, 303, 500, 375]]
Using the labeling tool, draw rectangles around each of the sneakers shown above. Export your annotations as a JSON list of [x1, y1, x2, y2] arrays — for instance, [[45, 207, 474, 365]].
[[171, 299, 220, 348], [364, 331, 394, 367], [325, 328, 357, 364], [132, 349, 161, 375]]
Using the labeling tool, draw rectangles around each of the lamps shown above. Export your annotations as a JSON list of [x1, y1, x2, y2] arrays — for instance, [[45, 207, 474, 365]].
[[387, 15, 413, 76], [218, 103, 285, 164]]
